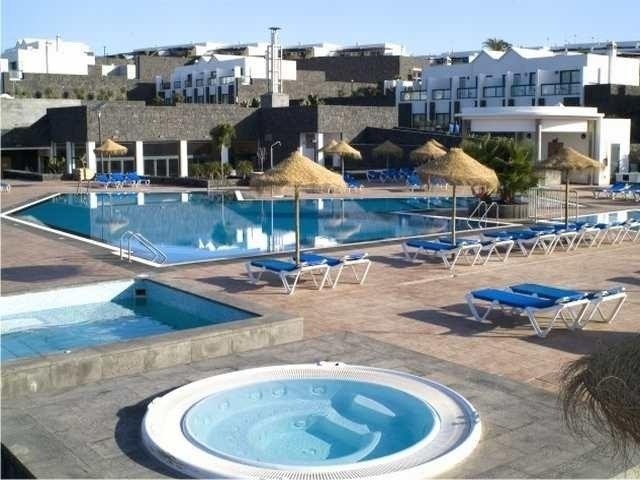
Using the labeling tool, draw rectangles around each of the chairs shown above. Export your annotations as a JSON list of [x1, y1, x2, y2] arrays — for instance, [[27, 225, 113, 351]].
[[590, 179, 640, 202], [343, 167, 449, 193], [94, 171, 151, 191], [465, 281, 628, 339], [401, 217, 640, 272], [244, 248, 373, 297]]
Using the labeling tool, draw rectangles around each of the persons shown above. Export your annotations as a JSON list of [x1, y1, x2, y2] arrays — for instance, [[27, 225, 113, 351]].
[[476, 187, 493, 217]]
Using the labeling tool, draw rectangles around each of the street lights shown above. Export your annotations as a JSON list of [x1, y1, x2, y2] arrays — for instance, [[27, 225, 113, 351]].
[[97, 110, 104, 176], [271, 141, 282, 168]]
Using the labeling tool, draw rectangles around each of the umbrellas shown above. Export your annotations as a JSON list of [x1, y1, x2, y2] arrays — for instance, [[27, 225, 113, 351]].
[[248, 149, 350, 267], [316, 140, 339, 153], [409, 140, 448, 191], [431, 138, 448, 151], [414, 147, 501, 247], [371, 140, 405, 169], [331, 141, 362, 161], [92, 138, 129, 172], [534, 143, 606, 232]]
